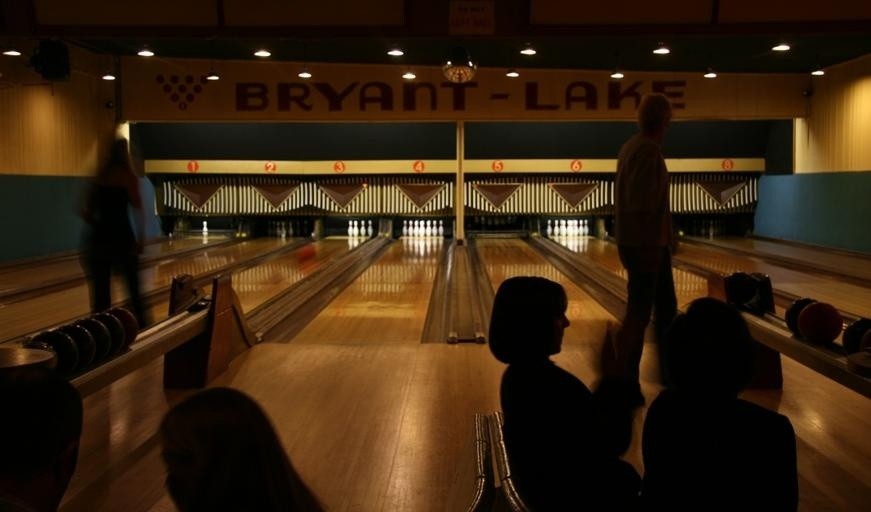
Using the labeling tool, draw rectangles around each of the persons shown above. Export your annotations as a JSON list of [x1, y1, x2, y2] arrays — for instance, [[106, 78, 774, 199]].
[[158, 385, 326, 511], [614, 92, 681, 409], [77, 132, 156, 331], [1, 374, 83, 512], [642, 294, 802, 511], [488, 275, 643, 511]]
